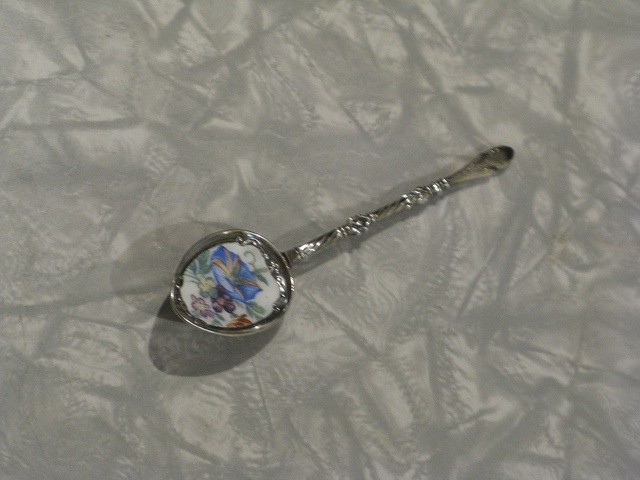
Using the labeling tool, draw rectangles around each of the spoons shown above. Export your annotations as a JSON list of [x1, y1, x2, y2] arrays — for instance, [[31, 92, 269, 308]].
[[165, 142, 515, 339]]
